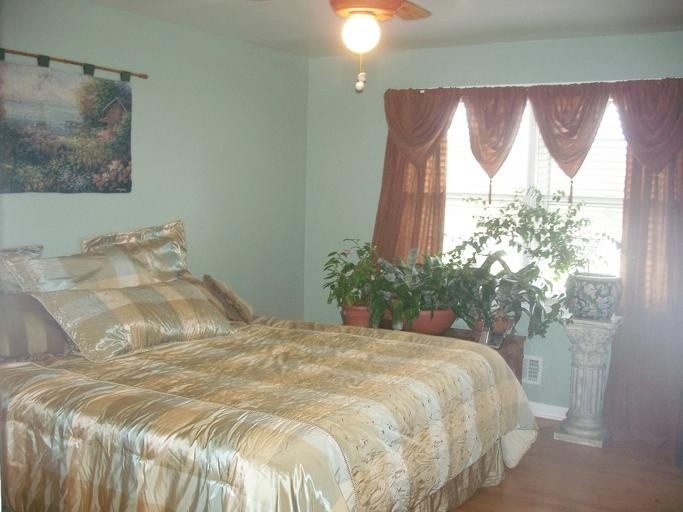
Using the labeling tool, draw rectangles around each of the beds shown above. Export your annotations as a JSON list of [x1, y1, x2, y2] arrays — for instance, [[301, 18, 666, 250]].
[[0, 219, 537, 512]]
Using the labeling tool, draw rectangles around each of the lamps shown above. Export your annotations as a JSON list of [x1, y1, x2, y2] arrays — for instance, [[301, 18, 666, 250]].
[[329, 0, 403, 94]]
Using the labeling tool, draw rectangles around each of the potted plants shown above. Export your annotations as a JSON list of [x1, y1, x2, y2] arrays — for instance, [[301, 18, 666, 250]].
[[321, 180, 622, 333]]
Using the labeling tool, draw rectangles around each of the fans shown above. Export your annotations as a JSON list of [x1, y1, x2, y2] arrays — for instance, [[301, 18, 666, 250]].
[[329, 0, 431, 21]]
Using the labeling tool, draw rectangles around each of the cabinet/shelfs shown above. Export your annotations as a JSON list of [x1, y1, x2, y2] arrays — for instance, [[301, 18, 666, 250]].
[[551, 314, 624, 448], [381, 322, 526, 385]]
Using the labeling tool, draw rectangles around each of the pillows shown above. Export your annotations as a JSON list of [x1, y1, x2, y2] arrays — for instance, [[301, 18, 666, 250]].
[[0, 219, 239, 360]]
